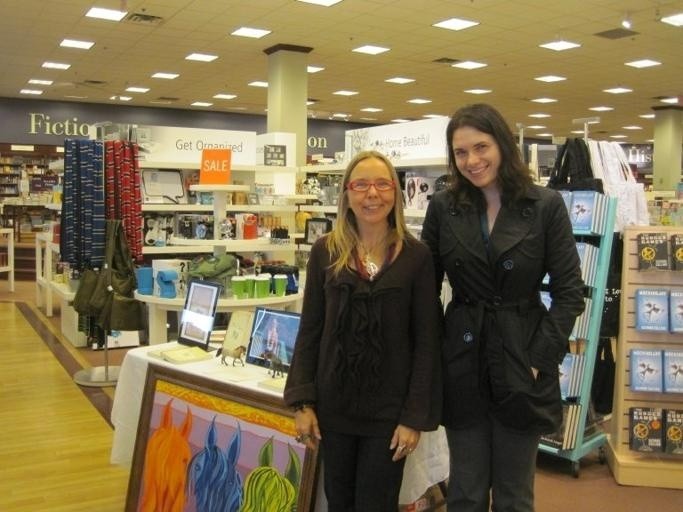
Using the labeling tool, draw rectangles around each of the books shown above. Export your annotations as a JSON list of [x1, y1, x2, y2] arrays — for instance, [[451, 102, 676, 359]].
[[147, 343, 213, 364], [557, 353, 584, 400], [571, 297, 592, 338], [574, 242, 599, 287], [627, 407, 683, 453], [629, 350, 683, 393], [557, 190, 607, 236], [535, 400, 581, 451], [635, 287, 682, 333], [637, 232, 683, 273]]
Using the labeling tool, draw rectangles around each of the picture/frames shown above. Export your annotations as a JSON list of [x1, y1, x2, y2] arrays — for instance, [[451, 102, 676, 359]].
[[246, 307, 302, 372], [178, 280, 220, 350], [124, 362, 321, 512]]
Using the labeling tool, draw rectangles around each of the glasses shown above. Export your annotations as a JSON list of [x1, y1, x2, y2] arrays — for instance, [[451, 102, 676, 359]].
[[346, 177, 396, 192]]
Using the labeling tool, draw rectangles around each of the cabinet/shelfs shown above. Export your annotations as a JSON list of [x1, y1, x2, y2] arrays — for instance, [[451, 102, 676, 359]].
[[0, 143, 65, 232], [601, 225, 682, 489], [36, 232, 91, 348], [138, 161, 299, 270], [301, 157, 453, 314], [538, 198, 617, 477], [134, 184, 304, 348]]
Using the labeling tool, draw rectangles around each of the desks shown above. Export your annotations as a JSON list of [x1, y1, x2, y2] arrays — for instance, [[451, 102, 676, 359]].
[[111, 340, 289, 467]]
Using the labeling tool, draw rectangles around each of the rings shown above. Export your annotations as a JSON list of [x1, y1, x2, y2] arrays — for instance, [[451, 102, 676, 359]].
[[398, 445, 406, 455], [301, 434, 311, 441], [407, 447, 412, 453], [293, 435, 303, 443]]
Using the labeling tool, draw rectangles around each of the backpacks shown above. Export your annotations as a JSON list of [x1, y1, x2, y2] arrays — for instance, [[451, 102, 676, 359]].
[[187, 254, 237, 294]]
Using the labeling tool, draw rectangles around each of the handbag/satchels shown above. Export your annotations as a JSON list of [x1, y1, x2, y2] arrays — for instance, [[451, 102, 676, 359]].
[[73, 267, 147, 331], [546, 137, 649, 232]]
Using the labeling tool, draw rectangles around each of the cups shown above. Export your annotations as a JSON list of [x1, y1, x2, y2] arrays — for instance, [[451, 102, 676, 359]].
[[274, 274, 288, 296], [231, 276, 245, 298], [258, 273, 272, 293], [246, 275, 255, 297], [255, 277, 269, 298]]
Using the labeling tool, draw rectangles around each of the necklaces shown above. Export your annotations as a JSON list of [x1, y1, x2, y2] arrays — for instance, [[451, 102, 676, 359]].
[[355, 229, 389, 276]]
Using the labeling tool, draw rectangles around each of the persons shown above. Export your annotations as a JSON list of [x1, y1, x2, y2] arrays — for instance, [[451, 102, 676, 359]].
[[285, 148, 437, 512], [420, 104, 586, 512]]
